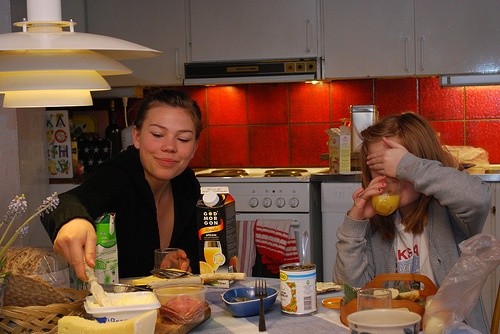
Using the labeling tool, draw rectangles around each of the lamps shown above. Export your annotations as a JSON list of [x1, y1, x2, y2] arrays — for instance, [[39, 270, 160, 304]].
[[0, 0, 161, 109]]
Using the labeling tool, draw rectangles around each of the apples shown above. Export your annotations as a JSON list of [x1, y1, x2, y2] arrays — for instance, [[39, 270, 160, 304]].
[[373, 287, 420, 301]]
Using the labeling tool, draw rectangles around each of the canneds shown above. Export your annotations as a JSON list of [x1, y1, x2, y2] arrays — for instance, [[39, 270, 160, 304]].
[[279, 262, 318, 317]]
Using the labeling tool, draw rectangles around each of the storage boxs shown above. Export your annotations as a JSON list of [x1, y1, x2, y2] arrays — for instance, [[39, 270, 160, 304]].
[[326, 126, 350, 174]]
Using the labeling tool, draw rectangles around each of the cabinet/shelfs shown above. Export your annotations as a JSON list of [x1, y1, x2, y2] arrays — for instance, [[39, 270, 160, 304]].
[[320, 181, 364, 283], [10, 0, 500, 98]]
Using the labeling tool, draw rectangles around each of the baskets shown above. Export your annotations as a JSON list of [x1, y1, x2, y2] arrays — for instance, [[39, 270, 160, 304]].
[[0, 271, 92, 334]]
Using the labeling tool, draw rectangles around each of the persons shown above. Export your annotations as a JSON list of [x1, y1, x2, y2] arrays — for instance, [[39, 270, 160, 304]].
[[332, 112, 492, 334], [43, 91, 203, 284]]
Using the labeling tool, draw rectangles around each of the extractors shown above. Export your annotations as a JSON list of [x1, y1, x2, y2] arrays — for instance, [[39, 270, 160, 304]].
[[182, 57, 322, 84]]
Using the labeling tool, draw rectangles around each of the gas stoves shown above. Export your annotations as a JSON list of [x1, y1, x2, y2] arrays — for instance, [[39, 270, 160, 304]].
[[194, 166, 330, 213]]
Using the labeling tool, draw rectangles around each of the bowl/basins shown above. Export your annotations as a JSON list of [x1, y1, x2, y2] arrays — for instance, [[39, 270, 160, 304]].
[[221, 286, 278, 317], [98, 283, 149, 293], [154, 284, 207, 324], [83, 291, 161, 324], [347, 309, 422, 334]]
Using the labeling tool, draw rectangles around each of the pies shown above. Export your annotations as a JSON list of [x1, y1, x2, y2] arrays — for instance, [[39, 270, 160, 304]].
[[0, 246, 68, 307]]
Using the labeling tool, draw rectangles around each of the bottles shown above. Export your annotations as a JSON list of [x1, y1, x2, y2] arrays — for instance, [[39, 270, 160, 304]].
[[105, 108, 122, 157]]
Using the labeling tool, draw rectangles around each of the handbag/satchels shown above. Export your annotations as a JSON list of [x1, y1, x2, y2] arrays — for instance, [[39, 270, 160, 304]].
[[422, 233, 500, 334]]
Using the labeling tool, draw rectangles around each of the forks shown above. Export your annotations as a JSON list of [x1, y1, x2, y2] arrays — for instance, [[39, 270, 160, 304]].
[[255, 278, 267, 332]]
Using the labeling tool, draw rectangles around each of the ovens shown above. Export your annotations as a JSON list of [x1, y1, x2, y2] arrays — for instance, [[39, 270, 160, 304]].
[[235, 213, 322, 280]]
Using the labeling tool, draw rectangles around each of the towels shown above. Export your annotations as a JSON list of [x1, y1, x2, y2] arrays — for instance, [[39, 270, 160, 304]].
[[236, 219, 257, 277], [254, 218, 300, 275]]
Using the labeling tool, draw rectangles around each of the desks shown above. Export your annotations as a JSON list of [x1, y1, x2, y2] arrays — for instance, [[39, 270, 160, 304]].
[[116, 277, 481, 334]]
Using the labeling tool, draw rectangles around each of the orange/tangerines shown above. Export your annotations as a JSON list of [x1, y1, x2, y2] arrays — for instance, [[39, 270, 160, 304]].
[[200, 261, 213, 273]]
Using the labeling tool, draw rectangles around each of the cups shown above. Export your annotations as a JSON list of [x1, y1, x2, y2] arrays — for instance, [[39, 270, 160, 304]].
[[154, 248, 181, 272], [372, 177, 403, 216], [357, 289, 392, 313], [280, 262, 317, 316], [203, 240, 222, 274]]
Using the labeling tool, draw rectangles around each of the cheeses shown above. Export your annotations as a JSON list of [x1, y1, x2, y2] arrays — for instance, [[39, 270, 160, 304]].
[[58, 310, 157, 334]]
